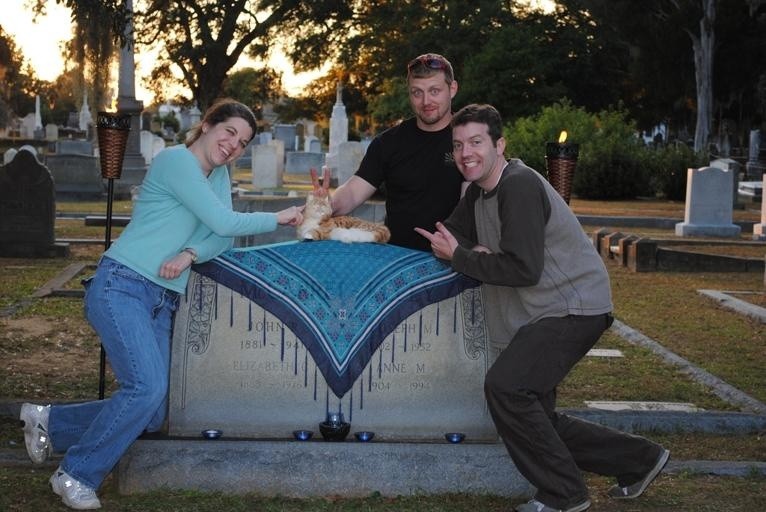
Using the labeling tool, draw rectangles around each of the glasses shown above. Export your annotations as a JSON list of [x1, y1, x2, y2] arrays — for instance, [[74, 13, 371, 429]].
[[406, 58, 446, 70]]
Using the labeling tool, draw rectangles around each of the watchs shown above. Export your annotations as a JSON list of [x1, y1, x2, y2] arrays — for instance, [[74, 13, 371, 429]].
[[183, 249, 196, 263]]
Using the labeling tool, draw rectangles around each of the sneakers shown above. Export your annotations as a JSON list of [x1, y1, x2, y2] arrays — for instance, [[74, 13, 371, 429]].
[[48, 465, 101, 511], [605, 446, 670, 501], [512, 499, 592, 512], [19, 403, 53, 465]]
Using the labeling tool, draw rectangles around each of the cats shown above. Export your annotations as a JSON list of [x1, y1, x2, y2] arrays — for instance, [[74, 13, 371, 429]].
[[296, 194, 393, 244]]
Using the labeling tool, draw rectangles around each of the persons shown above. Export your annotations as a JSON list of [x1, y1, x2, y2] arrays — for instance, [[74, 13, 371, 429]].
[[309, 54, 472, 253], [412, 104, 673, 511], [17, 97, 306, 510]]
